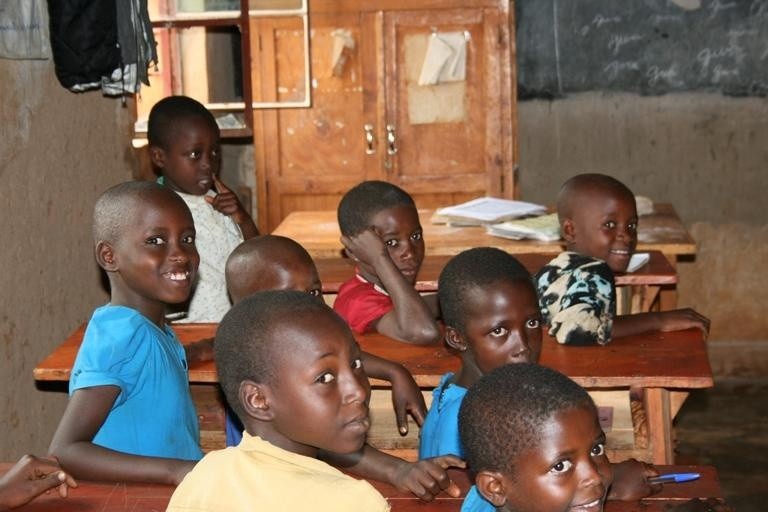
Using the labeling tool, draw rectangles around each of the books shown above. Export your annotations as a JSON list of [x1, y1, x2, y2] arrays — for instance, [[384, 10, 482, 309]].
[[625, 253, 650, 273], [432, 196, 564, 242]]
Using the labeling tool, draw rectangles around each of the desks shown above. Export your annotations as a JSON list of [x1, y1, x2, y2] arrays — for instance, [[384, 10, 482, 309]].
[[270, 202, 699, 314], [32, 321, 714, 465], [0, 461, 726, 511]]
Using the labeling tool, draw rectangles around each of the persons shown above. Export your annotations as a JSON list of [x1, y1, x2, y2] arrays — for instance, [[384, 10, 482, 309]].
[[225, 234, 468, 503], [533, 173, 712, 345], [146, 94, 259, 324], [165, 289, 393, 511], [47, 179, 198, 485], [0, 454, 78, 511], [460, 363, 663, 512], [332, 180, 442, 345], [418, 248, 543, 460]]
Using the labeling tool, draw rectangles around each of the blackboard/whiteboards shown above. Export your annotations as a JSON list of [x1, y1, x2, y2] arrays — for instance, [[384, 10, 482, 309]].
[[514, 0, 768, 101]]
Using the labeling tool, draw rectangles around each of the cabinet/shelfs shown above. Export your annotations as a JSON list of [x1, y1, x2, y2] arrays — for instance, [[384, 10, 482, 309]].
[[245, 1, 520, 230]]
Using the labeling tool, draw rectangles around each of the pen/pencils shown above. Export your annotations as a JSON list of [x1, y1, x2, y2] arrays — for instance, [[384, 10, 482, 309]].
[[646, 472, 702, 486]]
